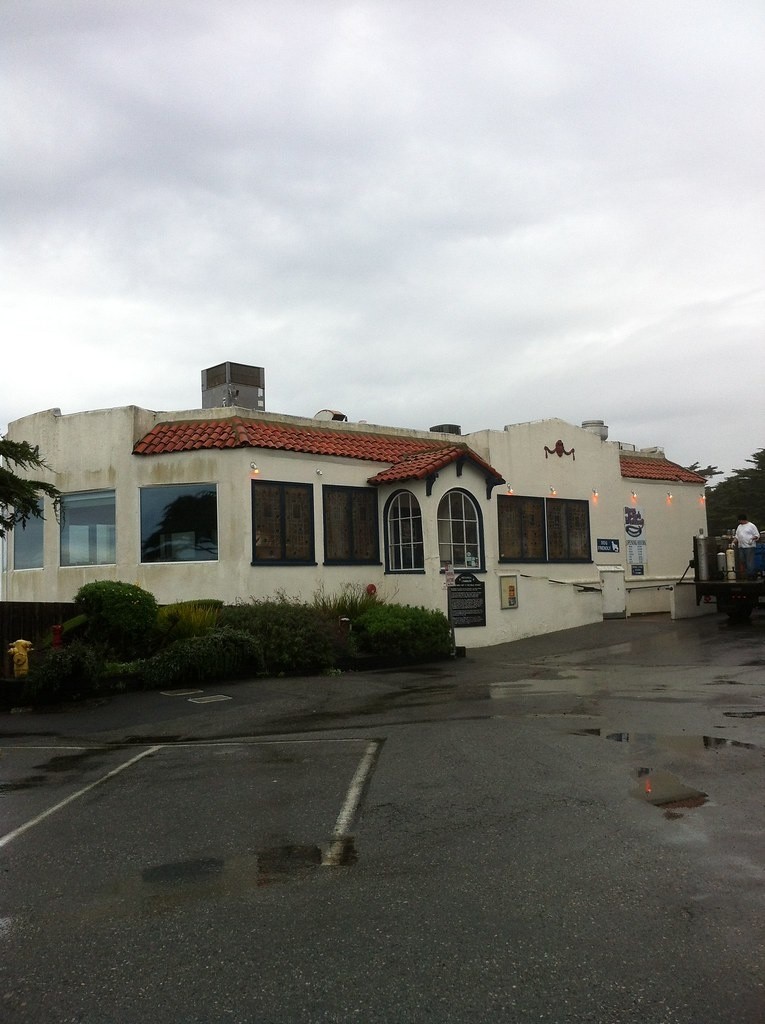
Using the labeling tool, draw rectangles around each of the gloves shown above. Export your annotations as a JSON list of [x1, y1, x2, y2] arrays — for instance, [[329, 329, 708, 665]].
[[731, 541, 735, 548]]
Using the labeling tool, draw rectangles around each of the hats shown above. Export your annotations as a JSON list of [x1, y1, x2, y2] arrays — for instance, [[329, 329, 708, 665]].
[[738, 514, 746, 520]]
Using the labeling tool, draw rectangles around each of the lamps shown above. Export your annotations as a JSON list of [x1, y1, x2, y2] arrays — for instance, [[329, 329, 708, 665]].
[[550, 486, 556, 496], [700, 494, 706, 500], [592, 488, 599, 497], [631, 490, 637, 498], [667, 491, 672, 499], [338, 616, 353, 632], [250, 462, 260, 474], [507, 483, 513, 494], [316, 469, 323, 476]]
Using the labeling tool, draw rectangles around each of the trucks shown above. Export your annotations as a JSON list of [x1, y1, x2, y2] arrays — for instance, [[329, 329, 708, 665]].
[[694, 535, 765, 620]]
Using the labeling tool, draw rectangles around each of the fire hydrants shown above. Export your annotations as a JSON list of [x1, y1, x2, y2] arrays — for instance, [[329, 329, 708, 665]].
[[8, 640, 34, 678], [52, 625, 65, 651]]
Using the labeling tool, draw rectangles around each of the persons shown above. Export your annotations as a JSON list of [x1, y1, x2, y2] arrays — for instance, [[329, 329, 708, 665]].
[[731, 514, 760, 580]]
